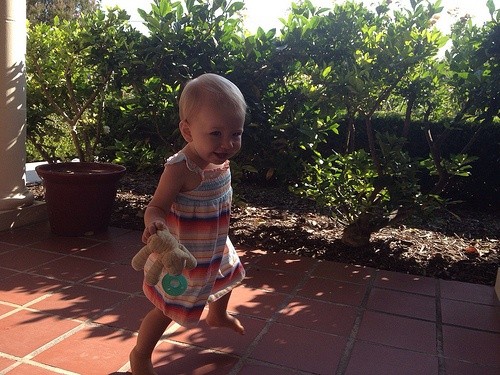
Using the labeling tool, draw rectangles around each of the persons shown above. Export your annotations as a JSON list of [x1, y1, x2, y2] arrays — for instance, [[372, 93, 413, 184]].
[[129, 73, 246, 375]]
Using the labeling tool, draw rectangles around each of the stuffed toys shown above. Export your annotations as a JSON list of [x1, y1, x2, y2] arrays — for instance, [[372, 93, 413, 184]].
[[131, 228, 198, 286]]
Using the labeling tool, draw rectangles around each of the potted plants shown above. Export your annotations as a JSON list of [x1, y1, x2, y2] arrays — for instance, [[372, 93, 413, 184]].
[[25, 5, 141, 236]]
